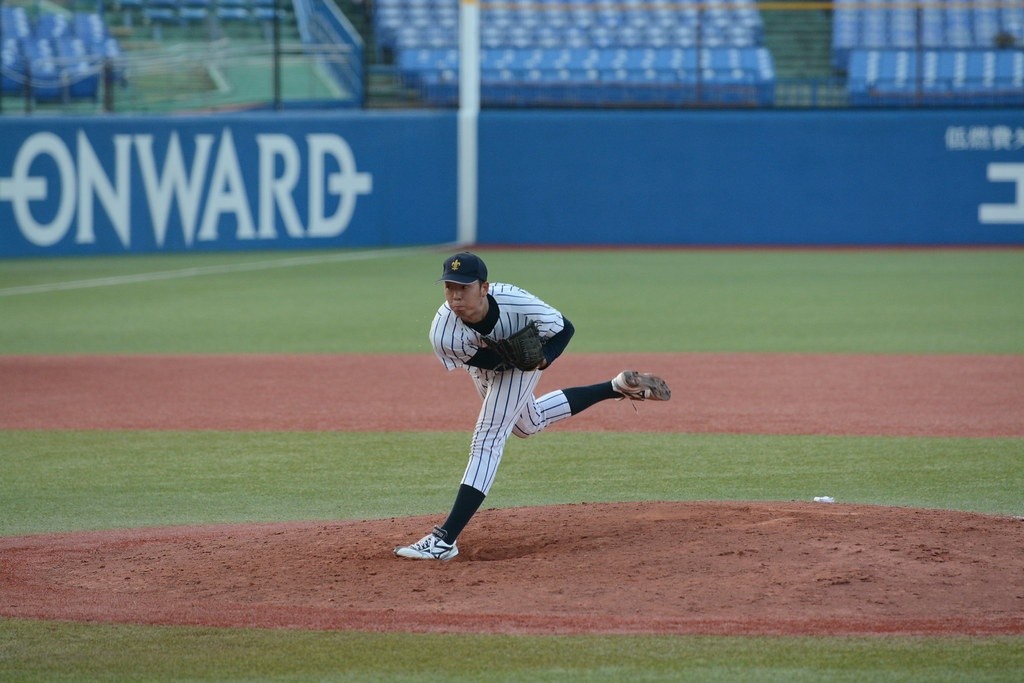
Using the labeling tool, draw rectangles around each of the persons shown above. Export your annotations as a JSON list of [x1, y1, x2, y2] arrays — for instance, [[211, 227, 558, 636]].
[[393, 252, 671, 560]]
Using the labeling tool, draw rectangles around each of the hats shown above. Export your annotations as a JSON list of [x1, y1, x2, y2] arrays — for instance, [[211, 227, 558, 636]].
[[434, 250, 488, 284]]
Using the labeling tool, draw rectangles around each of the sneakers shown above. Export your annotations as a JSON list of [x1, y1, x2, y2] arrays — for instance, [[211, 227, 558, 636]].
[[612, 369, 671, 415], [393, 526, 458, 560]]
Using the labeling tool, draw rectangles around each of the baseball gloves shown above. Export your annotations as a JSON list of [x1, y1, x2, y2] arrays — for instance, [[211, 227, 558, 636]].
[[480, 318, 545, 377]]
